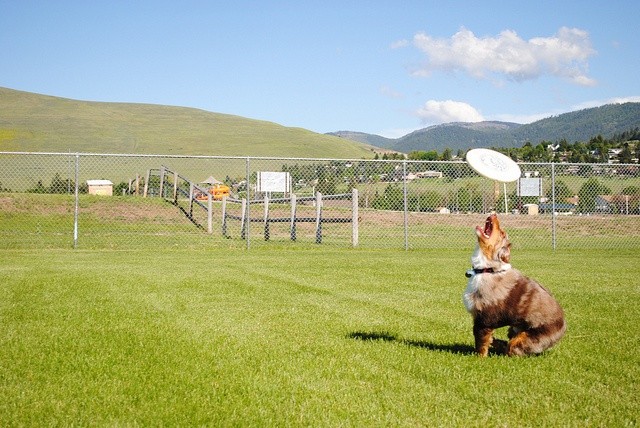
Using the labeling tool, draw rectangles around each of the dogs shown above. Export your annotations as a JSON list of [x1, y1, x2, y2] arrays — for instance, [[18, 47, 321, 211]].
[[462, 212, 567, 357]]
